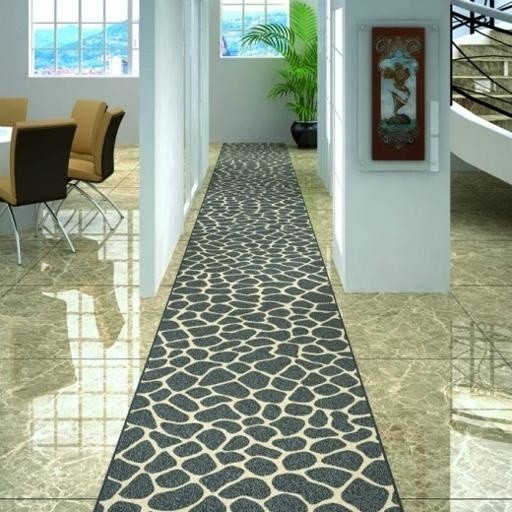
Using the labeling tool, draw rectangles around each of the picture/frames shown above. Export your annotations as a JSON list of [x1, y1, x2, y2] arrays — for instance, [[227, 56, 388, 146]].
[[371, 26, 427, 163]]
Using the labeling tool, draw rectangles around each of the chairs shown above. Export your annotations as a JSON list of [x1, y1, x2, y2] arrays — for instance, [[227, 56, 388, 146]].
[[2, 260, 127, 402], [0, 95, 126, 258]]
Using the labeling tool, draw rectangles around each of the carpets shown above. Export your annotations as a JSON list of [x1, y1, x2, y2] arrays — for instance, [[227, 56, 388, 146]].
[[95, 143, 399, 511]]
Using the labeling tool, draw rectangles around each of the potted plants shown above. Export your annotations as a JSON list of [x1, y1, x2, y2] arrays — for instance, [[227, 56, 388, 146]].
[[238, 1, 316, 151]]
